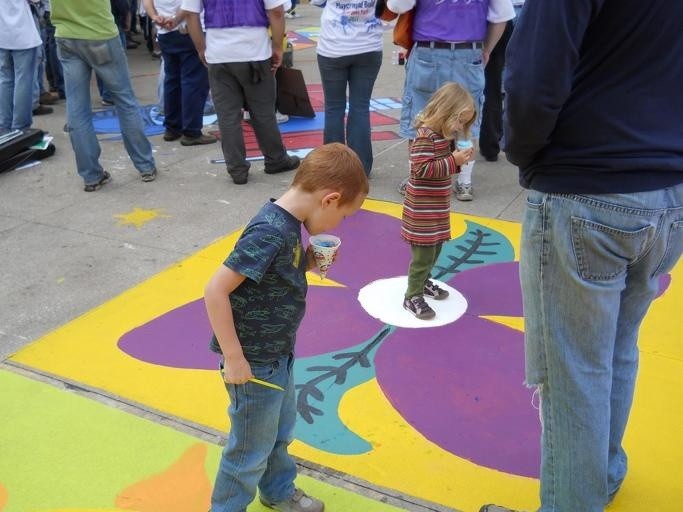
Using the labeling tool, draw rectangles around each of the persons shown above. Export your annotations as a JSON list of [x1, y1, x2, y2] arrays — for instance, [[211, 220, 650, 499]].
[[203, 142, 371, 511], [399, 81, 478, 322], [478, 2, 682, 512], [1, 0, 529, 203]]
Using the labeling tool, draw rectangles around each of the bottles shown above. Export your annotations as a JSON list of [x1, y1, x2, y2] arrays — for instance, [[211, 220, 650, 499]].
[[391, 44, 405, 66]]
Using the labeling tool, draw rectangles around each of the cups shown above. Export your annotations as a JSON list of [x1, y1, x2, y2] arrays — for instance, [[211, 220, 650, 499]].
[[309, 234, 341, 282]]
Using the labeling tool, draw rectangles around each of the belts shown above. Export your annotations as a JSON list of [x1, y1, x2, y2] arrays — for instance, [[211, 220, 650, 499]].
[[415, 39, 485, 51]]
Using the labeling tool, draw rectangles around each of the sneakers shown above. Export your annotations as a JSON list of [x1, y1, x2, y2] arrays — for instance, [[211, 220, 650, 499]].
[[141, 168, 156, 181], [479, 504, 518, 511], [259, 487, 325, 512], [275, 108, 290, 124], [403, 293, 436, 320], [480, 148, 500, 161], [397, 177, 409, 195], [163, 131, 178, 141], [451, 177, 473, 202], [83, 169, 112, 191], [181, 133, 218, 147], [28, 88, 65, 114], [421, 277, 449, 301], [233, 167, 248, 184], [242, 110, 250, 122], [126, 29, 161, 60], [101, 98, 114, 105], [264, 154, 300, 175]]
